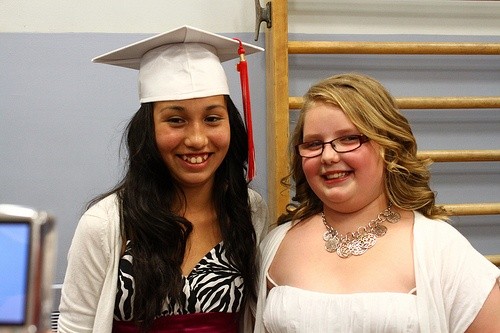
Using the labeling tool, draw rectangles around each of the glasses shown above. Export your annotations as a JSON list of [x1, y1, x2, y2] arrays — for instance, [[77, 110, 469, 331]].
[[294, 133, 370, 157]]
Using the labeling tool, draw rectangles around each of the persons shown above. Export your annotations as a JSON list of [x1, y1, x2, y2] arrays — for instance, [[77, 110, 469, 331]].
[[247, 72, 500, 333], [56, 24, 270, 333]]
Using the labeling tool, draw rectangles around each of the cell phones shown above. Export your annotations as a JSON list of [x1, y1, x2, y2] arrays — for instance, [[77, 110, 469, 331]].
[[0, 203, 57, 333]]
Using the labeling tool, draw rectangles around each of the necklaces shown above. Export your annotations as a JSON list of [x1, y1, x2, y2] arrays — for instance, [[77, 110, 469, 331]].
[[322, 201, 401, 259]]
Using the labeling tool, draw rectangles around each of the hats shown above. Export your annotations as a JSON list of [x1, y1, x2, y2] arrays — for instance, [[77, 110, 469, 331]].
[[90, 24, 265, 180]]
